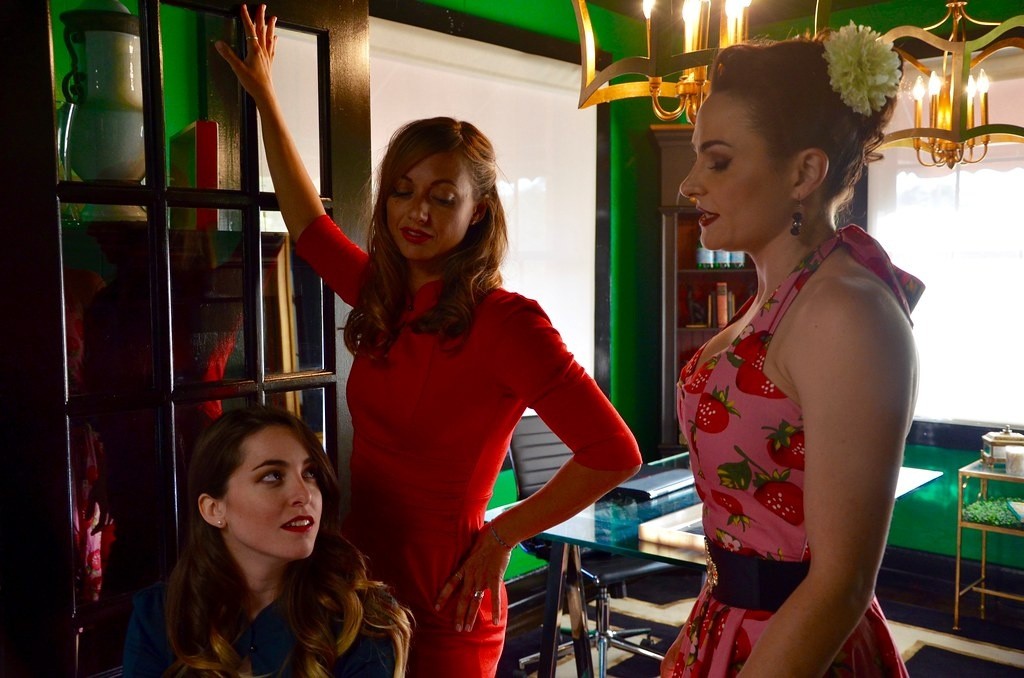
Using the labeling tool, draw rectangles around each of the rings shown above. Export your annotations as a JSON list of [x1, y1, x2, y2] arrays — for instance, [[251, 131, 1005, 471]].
[[245, 35, 260, 40], [454, 569, 463, 581], [473, 590, 485, 599]]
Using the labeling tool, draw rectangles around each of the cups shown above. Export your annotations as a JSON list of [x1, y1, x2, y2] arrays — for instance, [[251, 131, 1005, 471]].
[[715, 281, 728, 327]]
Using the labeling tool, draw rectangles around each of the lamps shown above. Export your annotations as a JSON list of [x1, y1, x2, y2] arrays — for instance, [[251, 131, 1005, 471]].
[[571, 1, 831, 131], [867, 1, 1023, 169]]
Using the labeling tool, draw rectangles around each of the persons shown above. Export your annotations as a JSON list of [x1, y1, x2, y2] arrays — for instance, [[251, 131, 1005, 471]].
[[122, 405, 414, 678], [213, 2, 644, 678], [659, 20, 920, 678], [7, 147, 303, 648]]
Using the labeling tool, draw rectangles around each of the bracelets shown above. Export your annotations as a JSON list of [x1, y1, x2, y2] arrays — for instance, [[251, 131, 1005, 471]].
[[490, 518, 518, 554]]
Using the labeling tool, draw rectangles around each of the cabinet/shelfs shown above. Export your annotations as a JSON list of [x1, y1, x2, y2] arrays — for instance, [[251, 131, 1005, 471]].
[[654, 204, 758, 461], [1, 1, 374, 678], [953, 459, 1023, 633]]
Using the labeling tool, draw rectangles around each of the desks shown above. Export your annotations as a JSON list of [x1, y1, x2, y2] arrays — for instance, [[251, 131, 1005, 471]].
[[483, 458, 944, 570]]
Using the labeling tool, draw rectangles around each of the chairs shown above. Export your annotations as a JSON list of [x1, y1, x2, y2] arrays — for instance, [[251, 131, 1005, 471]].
[[485, 444, 559, 678], [511, 415, 702, 678]]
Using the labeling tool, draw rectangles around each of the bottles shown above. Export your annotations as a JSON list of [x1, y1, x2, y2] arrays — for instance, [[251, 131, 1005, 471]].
[[698, 239, 745, 269]]
